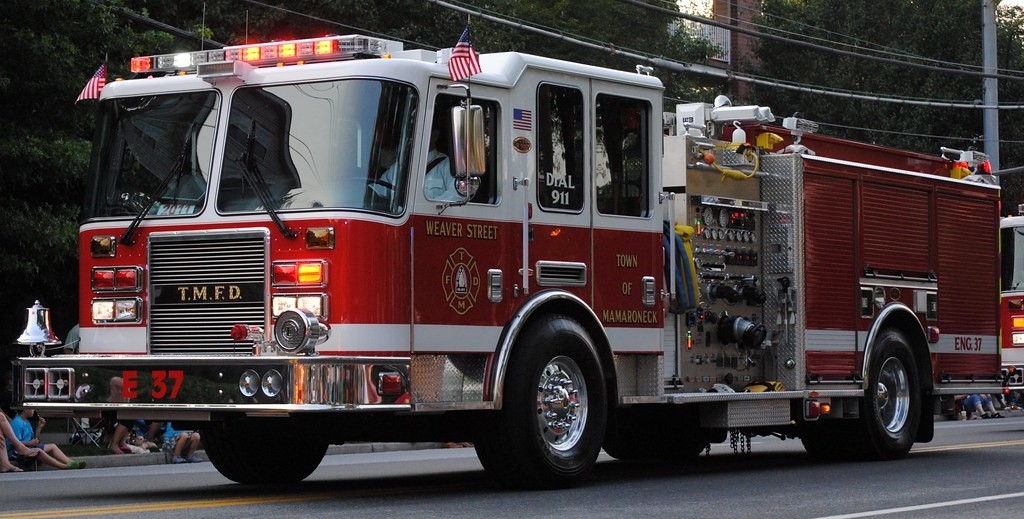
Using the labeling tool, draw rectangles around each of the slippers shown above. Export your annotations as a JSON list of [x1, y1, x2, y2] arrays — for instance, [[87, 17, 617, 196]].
[[0, 465, 24, 473], [16, 449, 41, 461]]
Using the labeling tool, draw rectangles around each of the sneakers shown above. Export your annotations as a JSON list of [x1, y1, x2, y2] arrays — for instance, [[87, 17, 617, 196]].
[[980, 413, 991, 419], [991, 412, 1005, 418]]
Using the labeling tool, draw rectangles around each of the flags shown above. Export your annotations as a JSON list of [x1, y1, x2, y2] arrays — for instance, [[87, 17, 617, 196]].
[[449, 26, 481, 82]]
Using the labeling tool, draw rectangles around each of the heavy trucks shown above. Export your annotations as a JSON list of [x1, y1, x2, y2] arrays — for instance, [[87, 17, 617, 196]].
[[9, 28, 1008, 489]]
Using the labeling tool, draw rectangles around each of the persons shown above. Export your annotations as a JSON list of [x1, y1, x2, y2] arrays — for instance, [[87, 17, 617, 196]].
[[367, 120, 460, 213], [953, 393, 1012, 420], [0, 408, 86, 472], [109, 419, 203, 462]]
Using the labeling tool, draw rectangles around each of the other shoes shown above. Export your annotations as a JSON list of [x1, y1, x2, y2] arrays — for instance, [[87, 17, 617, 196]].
[[1000, 404, 1011, 410], [967, 413, 978, 419], [111, 449, 124, 454], [1016, 406, 1022, 410], [66, 459, 86, 469], [119, 444, 132, 453], [171, 456, 188, 463], [957, 416, 962, 420], [185, 453, 203, 463]]
